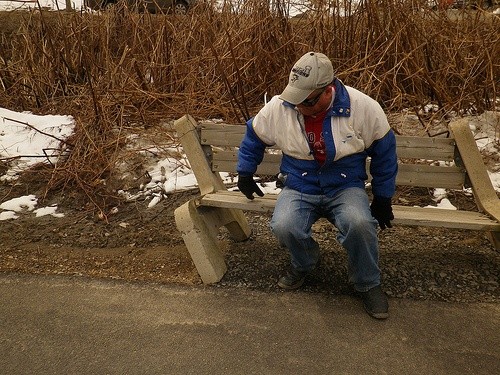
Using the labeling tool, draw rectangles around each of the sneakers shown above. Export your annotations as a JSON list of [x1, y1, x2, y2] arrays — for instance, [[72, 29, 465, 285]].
[[362, 286, 389, 319], [278, 260, 321, 289]]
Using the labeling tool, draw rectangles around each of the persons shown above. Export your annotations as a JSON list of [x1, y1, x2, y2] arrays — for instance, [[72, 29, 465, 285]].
[[237, 51, 398, 319]]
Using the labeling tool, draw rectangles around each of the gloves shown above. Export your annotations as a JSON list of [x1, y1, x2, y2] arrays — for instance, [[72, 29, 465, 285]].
[[237, 176, 264, 200], [370, 197, 395, 231]]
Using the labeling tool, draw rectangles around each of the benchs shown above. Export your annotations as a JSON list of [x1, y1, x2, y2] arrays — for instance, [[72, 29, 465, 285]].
[[173, 115, 500, 285]]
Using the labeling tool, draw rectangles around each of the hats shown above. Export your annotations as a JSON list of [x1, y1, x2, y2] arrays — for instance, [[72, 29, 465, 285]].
[[278, 51, 334, 105]]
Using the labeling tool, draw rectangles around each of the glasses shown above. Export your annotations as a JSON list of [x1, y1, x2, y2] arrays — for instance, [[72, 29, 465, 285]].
[[300, 87, 325, 107]]
